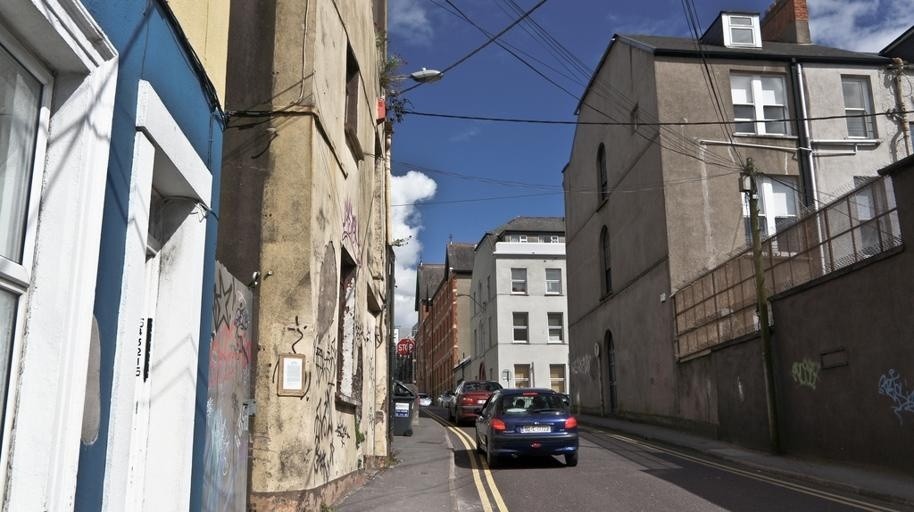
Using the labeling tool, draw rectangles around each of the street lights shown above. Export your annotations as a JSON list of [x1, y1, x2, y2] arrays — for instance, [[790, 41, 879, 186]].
[[380, 67, 444, 87]]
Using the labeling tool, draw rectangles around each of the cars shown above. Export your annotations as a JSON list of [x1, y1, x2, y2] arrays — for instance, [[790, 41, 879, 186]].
[[474, 388, 578, 467], [438, 380, 503, 424], [419, 393, 432, 406]]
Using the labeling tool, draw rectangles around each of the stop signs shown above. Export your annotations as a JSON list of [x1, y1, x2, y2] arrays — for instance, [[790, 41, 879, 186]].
[[398, 339, 415, 354]]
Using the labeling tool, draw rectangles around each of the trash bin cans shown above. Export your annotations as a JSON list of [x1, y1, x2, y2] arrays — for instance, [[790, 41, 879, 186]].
[[391, 379, 417, 437]]
